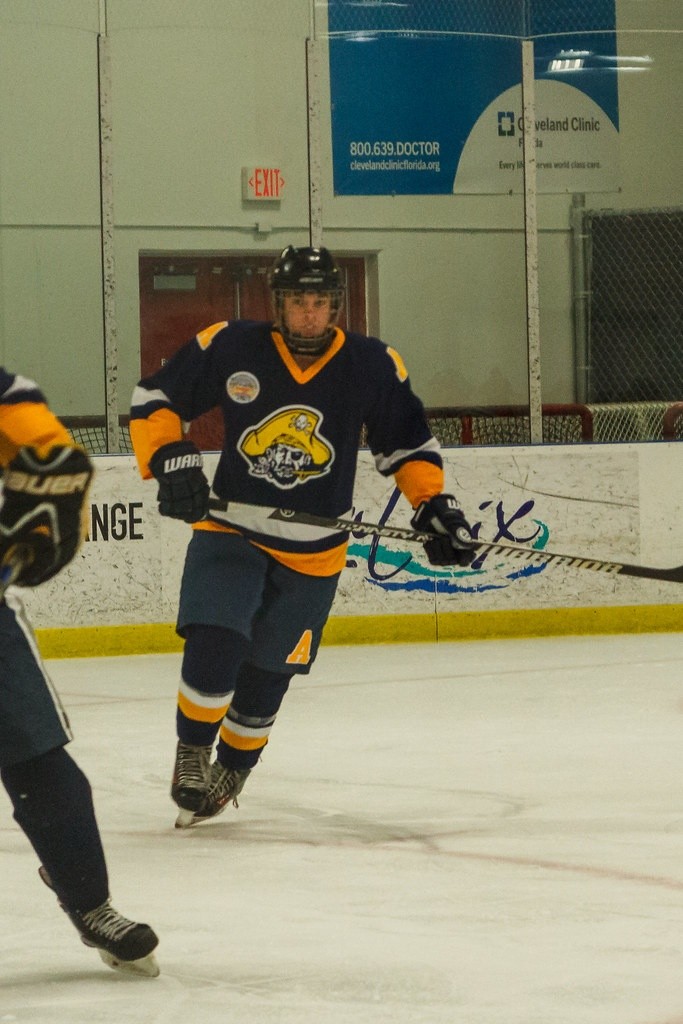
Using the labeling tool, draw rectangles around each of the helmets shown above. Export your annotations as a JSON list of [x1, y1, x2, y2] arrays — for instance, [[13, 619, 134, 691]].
[[267, 245, 345, 353]]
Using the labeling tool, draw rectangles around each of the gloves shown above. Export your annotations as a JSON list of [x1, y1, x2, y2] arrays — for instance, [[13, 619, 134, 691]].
[[411, 493, 476, 567], [148, 440, 210, 523], [0, 444, 93, 587]]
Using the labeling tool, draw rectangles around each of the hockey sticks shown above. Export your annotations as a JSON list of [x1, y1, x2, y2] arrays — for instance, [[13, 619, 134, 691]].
[[208, 497, 683, 585]]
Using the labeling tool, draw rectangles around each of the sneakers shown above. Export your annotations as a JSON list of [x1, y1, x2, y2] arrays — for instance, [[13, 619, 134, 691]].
[[187, 758, 252, 825], [38, 866, 160, 977], [171, 742, 214, 828]]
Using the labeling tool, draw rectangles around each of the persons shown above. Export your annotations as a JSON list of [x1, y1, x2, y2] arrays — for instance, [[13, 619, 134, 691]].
[[0, 363, 160, 976], [130, 244, 479, 829]]
